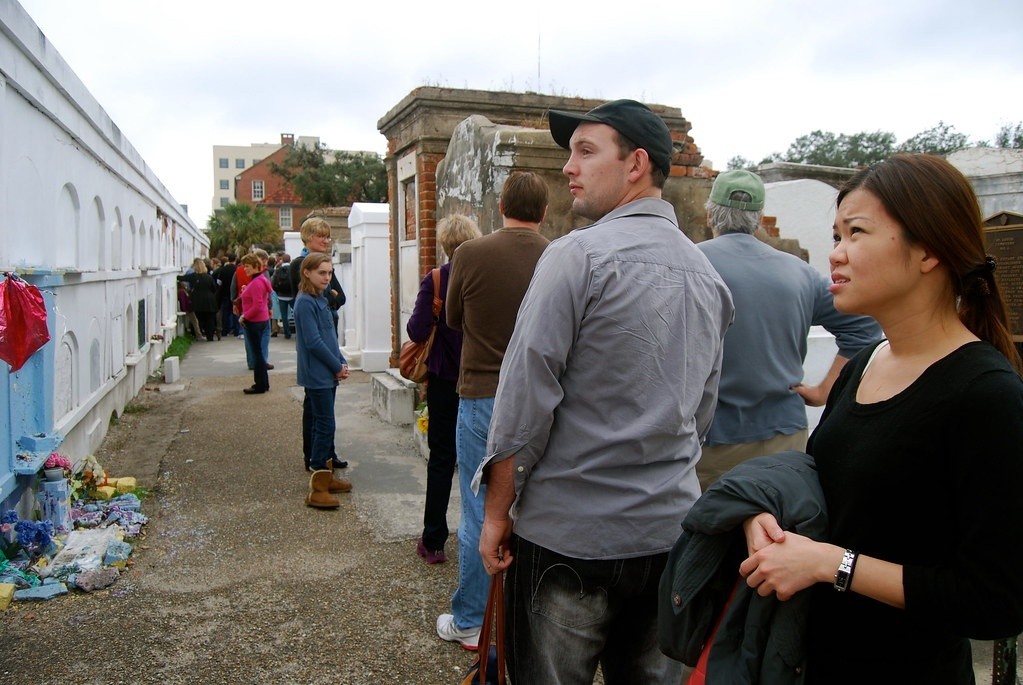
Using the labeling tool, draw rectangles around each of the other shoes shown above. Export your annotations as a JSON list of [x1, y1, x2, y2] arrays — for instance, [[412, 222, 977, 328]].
[[333, 458, 347, 468], [251, 385, 269, 391], [249, 363, 274, 370], [244, 387, 266, 394]]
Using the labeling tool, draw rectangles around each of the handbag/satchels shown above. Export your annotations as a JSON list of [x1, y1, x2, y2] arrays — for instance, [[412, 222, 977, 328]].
[[398, 268, 443, 382], [462, 569, 506, 685]]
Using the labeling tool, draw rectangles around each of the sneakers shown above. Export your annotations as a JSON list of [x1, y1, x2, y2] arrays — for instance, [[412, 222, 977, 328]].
[[417, 537, 445, 563], [437, 613, 481, 650]]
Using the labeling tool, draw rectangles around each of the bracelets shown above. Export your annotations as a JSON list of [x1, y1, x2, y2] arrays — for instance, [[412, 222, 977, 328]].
[[846, 551, 860, 590]]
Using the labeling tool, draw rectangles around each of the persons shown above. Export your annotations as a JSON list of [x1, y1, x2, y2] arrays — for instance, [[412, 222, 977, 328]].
[[289, 217, 348, 472], [233, 253, 273, 394], [407, 213, 482, 565], [470, 99, 736, 685], [293, 253, 353, 508], [437, 171, 550, 650], [738, 153, 1023, 685], [694, 170, 883, 495], [177, 245, 295, 342], [231, 248, 274, 370]]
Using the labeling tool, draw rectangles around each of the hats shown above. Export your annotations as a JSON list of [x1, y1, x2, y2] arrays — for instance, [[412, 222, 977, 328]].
[[548, 100, 672, 178], [710, 169, 765, 211]]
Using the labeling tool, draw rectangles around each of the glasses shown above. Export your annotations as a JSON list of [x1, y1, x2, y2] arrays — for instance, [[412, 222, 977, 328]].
[[317, 235, 331, 241]]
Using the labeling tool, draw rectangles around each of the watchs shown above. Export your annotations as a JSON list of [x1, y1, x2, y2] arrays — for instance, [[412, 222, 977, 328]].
[[834, 549, 855, 592]]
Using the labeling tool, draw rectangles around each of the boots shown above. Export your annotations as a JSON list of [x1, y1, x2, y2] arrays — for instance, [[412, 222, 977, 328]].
[[310, 457, 352, 493], [305, 467, 339, 507]]
